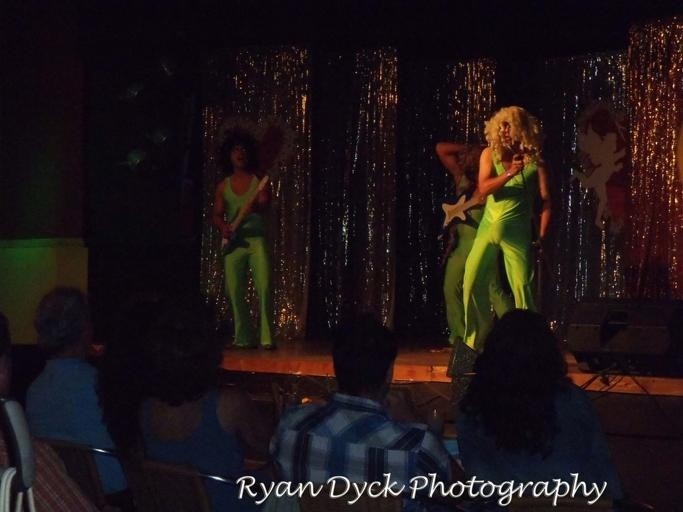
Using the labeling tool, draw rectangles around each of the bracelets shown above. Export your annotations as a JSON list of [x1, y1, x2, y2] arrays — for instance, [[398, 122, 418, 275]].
[[504, 170, 513, 179]]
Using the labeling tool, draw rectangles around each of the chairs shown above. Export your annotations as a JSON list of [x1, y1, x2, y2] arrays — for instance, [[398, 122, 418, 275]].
[[0, 400, 627, 507]]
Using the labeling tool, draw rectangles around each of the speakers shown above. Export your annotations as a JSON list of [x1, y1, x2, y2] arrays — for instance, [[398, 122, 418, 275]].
[[568, 297, 683, 379]]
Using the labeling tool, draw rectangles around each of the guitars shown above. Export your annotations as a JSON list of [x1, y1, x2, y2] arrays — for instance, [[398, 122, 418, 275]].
[[441, 192, 477, 229], [212, 176, 271, 255]]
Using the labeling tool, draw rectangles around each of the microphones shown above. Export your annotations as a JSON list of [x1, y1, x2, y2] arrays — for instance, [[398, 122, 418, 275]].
[[512, 139, 521, 161]]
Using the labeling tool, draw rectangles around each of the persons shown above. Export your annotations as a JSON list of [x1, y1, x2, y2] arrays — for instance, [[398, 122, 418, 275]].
[[434, 139, 517, 358], [461, 102, 556, 355], [1, 283, 631, 510], [210, 125, 279, 351]]
